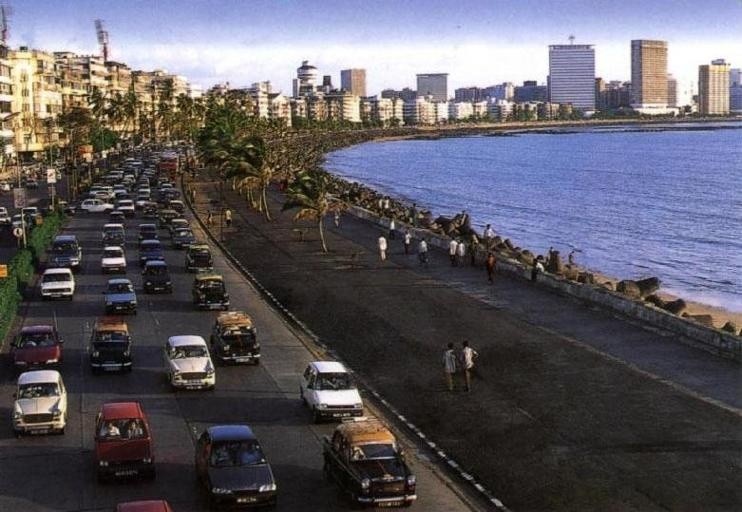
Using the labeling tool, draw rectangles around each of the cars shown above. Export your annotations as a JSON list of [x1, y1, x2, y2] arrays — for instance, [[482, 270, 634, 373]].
[[102, 277, 139, 316], [9, 325, 67, 376], [38, 267, 76, 302], [159, 333, 217, 392], [194, 423, 281, 512], [0, 145, 199, 273], [299, 360, 364, 425], [321, 418, 418, 510], [115, 498, 172, 511]]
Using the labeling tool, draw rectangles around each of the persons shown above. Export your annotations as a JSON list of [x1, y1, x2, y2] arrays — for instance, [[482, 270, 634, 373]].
[[328, 376, 340, 389], [239, 443, 261, 467], [190, 187, 197, 207], [333, 207, 341, 229], [223, 208, 231, 228], [121, 419, 144, 440], [343, 445, 366, 460], [206, 209, 212, 229], [530, 258, 538, 283], [458, 340, 480, 393], [377, 219, 497, 285], [212, 445, 232, 466], [440, 341, 457, 393], [99, 421, 120, 436]]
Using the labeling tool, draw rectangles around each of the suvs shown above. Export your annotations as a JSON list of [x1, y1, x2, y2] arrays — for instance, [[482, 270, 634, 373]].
[[212, 308, 262, 366], [94, 398, 156, 484], [87, 315, 134, 375], [142, 258, 175, 295], [185, 243, 216, 274], [192, 270, 231, 313], [13, 368, 69, 436]]
